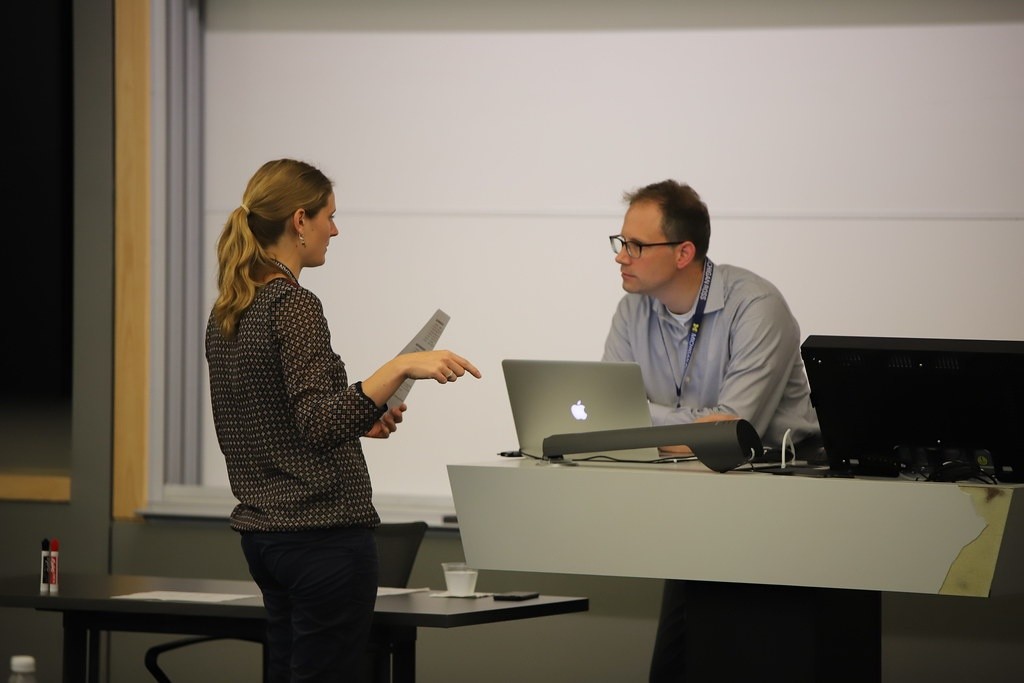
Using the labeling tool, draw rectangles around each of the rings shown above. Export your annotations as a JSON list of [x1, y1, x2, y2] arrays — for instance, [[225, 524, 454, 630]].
[[447, 371, 452, 378]]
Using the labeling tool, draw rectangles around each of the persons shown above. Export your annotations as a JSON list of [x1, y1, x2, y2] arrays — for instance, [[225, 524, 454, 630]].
[[600, 180, 828, 467], [204, 160, 482, 683]]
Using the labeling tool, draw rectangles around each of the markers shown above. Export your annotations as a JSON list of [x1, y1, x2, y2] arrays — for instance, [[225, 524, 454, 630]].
[[40, 538, 49, 592], [50, 538, 58, 592]]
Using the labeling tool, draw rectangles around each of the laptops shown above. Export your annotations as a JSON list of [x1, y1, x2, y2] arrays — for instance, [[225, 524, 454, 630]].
[[502, 360, 698, 462]]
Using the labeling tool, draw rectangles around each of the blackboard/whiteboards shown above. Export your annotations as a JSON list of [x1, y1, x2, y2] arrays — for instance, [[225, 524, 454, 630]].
[[197, 0, 1021, 498]]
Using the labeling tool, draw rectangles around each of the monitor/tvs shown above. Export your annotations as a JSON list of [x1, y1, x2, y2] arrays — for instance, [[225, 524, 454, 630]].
[[801, 334, 1024, 484]]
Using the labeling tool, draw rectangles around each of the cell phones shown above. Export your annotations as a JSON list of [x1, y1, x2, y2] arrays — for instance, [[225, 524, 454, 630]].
[[494, 592, 539, 602]]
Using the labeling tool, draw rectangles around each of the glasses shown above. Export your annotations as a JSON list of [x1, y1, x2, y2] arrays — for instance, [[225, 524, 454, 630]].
[[610, 235, 685, 258]]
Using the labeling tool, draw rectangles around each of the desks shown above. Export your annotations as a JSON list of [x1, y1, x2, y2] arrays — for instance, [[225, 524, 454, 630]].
[[0, 575, 589, 683]]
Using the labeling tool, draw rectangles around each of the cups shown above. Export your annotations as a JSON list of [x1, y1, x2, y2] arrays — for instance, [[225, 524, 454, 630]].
[[441, 562, 479, 596]]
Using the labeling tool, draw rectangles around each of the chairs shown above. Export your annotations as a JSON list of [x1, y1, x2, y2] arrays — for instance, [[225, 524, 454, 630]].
[[145, 522, 427, 683]]
